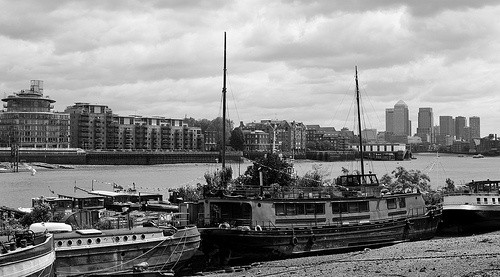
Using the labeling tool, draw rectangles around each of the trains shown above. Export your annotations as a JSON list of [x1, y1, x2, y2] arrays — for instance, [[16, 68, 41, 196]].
[[0, 146, 85, 154]]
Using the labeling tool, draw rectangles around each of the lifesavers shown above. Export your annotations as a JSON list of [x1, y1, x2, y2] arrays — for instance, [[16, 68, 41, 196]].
[[255, 225, 263, 231]]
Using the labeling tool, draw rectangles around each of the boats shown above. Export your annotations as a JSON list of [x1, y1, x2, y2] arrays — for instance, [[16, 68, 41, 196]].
[[0, 229, 56, 277], [433, 178, 500, 237], [17, 182, 202, 277], [168, 30, 443, 260]]
